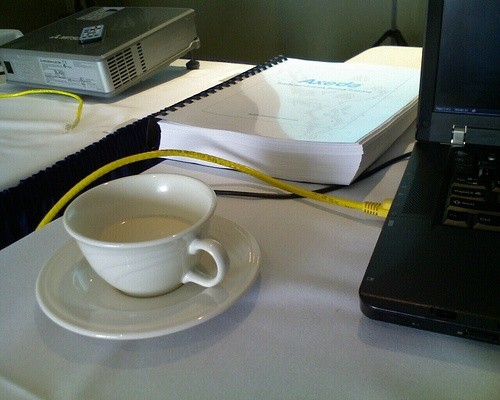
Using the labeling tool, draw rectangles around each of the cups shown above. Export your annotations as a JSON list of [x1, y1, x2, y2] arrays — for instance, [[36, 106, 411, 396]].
[[62, 172, 230, 298]]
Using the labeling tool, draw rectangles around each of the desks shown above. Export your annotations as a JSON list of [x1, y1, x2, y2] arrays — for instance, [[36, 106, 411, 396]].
[[0, 58, 500, 400]]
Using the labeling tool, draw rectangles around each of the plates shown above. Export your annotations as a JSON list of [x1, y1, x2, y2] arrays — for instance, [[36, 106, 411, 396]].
[[35, 216, 262, 340]]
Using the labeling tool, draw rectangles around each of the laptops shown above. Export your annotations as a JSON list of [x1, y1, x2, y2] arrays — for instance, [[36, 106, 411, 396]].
[[359, 0, 500, 345]]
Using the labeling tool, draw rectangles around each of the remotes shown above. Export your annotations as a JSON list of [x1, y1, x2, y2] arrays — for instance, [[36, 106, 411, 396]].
[[79, 25, 104, 43]]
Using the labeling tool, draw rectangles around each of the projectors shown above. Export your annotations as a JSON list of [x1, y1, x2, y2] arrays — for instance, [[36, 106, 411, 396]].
[[0, 6, 200, 97]]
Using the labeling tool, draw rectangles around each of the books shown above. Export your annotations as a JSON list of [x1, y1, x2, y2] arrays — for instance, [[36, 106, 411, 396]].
[[155, 54, 423, 186]]
